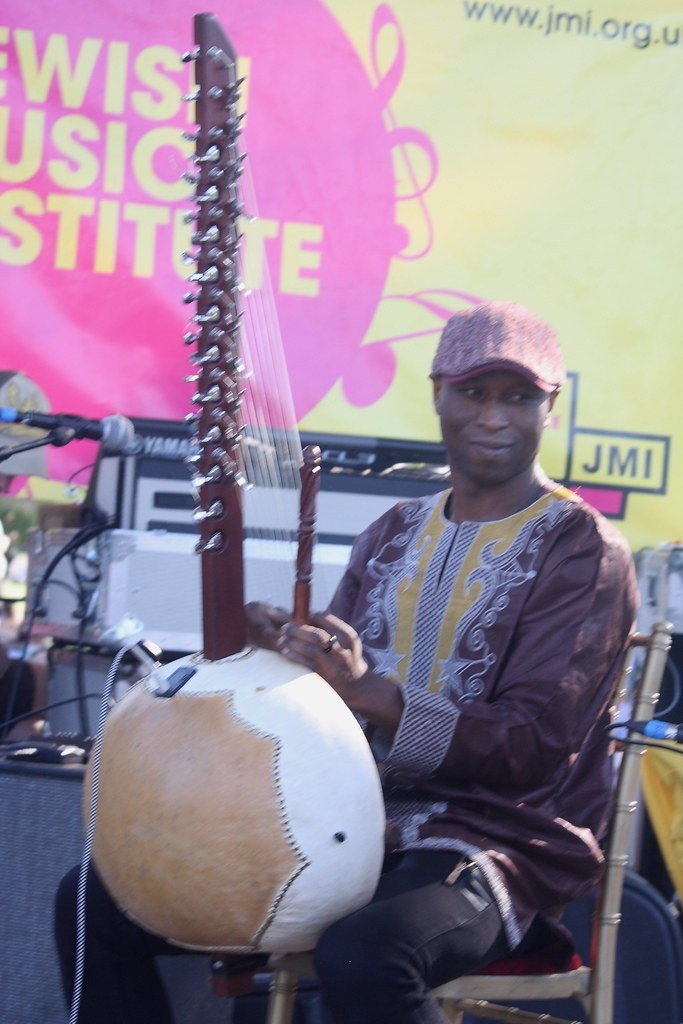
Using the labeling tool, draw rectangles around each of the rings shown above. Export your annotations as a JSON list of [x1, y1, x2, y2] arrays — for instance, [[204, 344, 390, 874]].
[[323, 636, 338, 654]]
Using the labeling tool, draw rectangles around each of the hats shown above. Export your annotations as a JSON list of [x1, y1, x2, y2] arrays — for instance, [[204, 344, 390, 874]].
[[428, 299, 568, 394], [0, 369, 52, 477]]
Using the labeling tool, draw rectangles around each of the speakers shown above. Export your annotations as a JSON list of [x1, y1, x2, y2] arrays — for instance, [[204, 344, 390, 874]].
[[458, 868, 683, 1024], [0, 756, 318, 1024]]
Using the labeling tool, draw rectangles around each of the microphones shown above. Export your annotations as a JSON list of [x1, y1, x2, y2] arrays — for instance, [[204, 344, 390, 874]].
[[0, 406, 136, 456]]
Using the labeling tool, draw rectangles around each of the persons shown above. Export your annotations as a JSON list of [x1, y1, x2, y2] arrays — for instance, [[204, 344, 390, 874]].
[[54, 305, 643, 1024], [0, 372, 51, 743]]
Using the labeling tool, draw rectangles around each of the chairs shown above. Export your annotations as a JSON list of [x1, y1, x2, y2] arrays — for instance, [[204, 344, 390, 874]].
[[267, 620, 673, 1024]]
